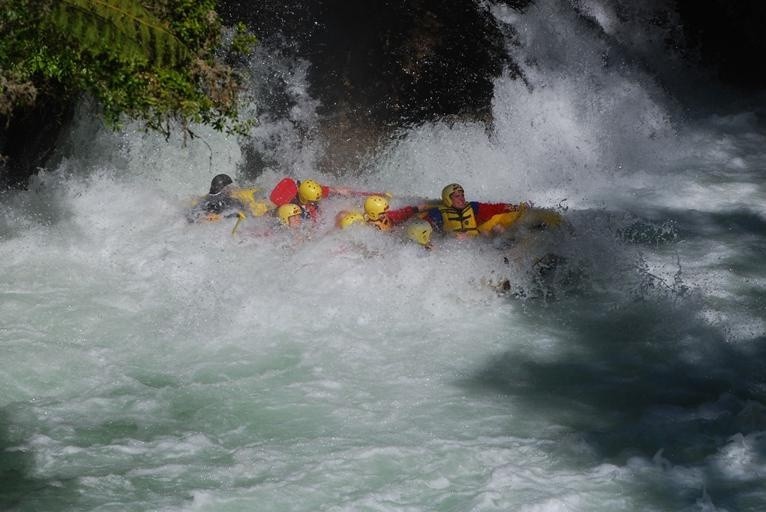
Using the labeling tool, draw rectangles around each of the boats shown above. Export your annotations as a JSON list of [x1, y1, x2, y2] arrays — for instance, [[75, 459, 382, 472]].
[[186, 175, 562, 294]]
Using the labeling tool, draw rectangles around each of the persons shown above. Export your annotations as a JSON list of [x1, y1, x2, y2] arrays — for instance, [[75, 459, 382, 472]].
[[184, 174, 531, 248]]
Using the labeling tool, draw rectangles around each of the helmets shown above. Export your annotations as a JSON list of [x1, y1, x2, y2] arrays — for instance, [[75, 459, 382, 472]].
[[363, 195, 389, 221], [440, 182, 466, 207], [297, 178, 323, 207], [340, 212, 364, 230], [208, 173, 235, 197], [278, 203, 302, 227], [405, 218, 434, 245]]
